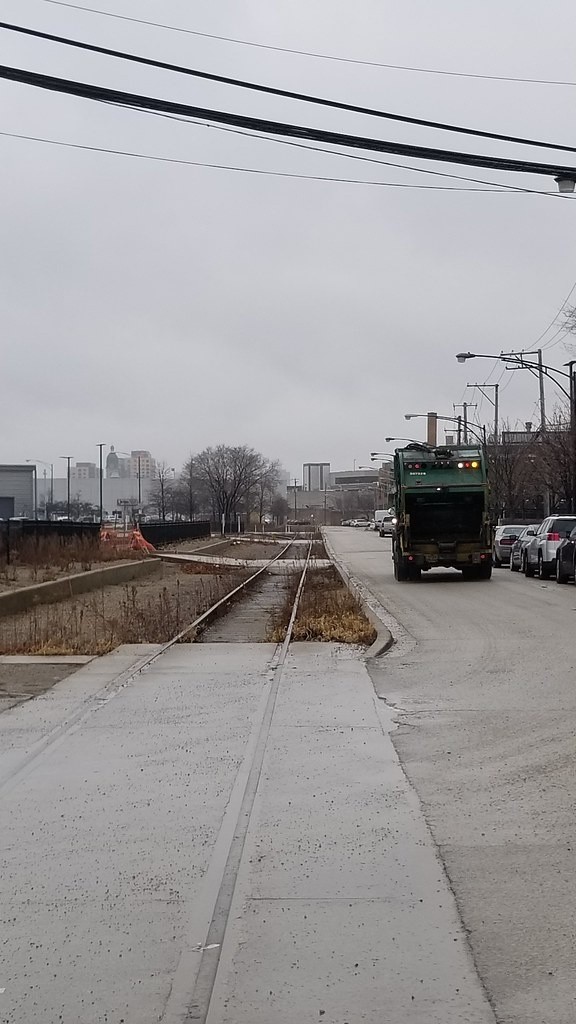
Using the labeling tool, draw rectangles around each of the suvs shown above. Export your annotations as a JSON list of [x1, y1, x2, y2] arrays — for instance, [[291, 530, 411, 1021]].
[[522, 513, 576, 580]]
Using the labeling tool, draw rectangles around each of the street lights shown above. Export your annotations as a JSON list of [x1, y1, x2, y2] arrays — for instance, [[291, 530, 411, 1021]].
[[405, 414, 487, 453], [455, 352, 576, 497]]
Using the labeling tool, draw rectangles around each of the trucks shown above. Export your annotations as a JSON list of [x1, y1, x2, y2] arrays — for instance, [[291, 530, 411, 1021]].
[[374, 509, 390, 531], [390, 444, 495, 582]]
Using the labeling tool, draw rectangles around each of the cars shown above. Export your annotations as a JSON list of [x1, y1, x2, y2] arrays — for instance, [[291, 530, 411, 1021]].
[[508, 524, 542, 571], [378, 515, 396, 537], [554, 526, 575, 584], [353, 519, 371, 527], [494, 524, 529, 568]]
